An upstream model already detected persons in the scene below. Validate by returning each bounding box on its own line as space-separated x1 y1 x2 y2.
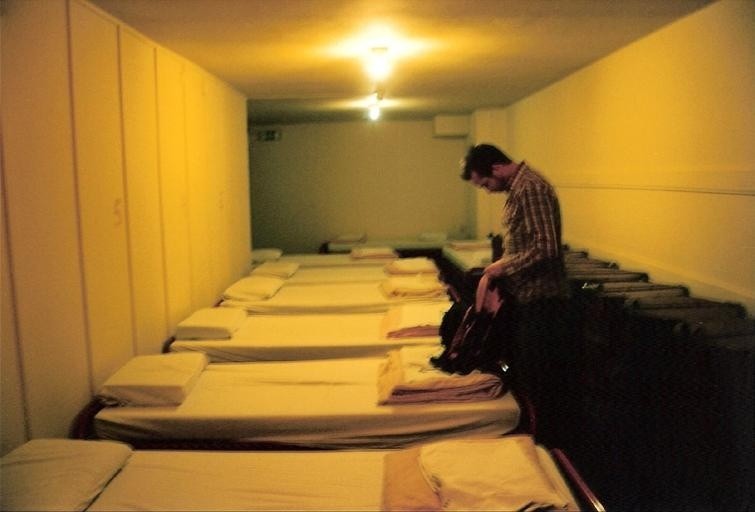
457 142 574 449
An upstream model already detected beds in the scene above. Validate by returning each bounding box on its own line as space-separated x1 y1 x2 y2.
94 344 537 450
251 245 399 268
252 256 441 286
170 301 455 362
219 274 460 315
1 435 603 512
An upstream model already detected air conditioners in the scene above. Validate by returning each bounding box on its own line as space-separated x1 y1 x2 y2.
431 114 470 137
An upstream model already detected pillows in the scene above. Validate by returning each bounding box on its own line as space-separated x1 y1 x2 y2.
174 307 247 340
98 352 211 407
1 439 132 512
251 261 299 279
222 276 283 303
252 248 283 262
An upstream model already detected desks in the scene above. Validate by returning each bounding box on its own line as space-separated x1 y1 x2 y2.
440 244 494 301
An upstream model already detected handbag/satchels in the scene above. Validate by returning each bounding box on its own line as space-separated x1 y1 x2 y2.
430 268 509 375
